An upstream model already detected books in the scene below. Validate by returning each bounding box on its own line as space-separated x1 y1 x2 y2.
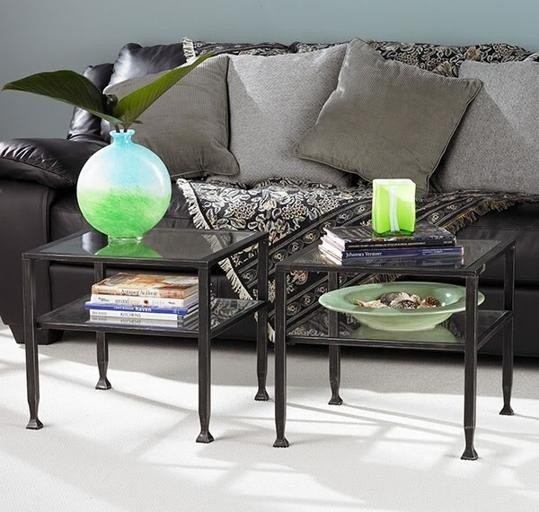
322 224 456 251
320 250 465 268
86 315 220 333
317 237 465 259
84 272 217 321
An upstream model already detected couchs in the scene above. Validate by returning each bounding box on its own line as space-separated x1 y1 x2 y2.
0 40 539 362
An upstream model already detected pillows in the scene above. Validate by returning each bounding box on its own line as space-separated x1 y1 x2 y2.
298 39 482 197
209 44 353 188
100 55 240 180
434 59 539 197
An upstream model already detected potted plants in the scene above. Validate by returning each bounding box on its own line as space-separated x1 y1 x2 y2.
0 53 219 257
